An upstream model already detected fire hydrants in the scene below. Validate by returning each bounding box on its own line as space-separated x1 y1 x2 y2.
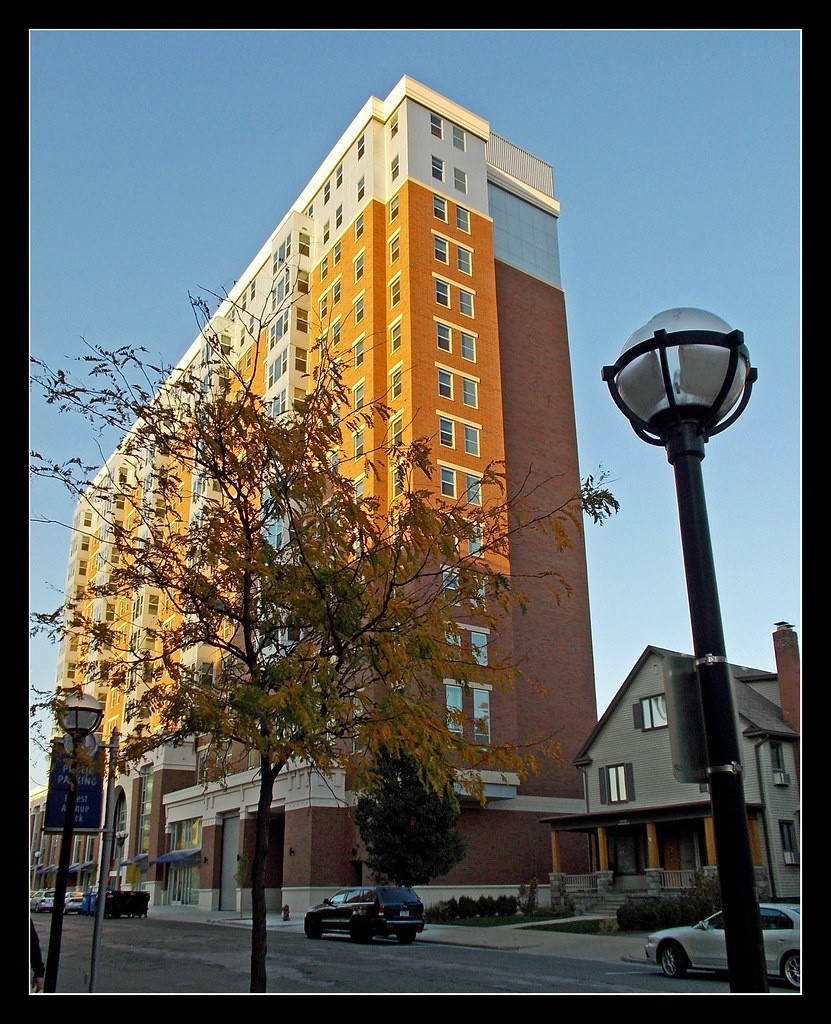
281 904 291 921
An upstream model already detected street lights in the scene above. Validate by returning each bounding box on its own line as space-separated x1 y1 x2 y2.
600 307 772 993
42 691 103 993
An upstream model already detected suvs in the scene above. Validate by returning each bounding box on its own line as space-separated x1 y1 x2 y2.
304 885 426 944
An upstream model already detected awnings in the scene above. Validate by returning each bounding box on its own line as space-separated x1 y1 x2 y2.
68 861 94 873
131 852 149 862
68 862 80 871
29 864 62 875
119 859 132 866
149 848 202 866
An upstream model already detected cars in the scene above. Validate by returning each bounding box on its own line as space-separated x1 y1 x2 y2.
644 903 800 991
30 891 83 915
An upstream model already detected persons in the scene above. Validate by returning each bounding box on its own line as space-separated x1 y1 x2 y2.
29 917 46 993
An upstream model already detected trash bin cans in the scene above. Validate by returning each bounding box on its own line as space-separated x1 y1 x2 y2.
82 891 97 917
112 890 150 918
